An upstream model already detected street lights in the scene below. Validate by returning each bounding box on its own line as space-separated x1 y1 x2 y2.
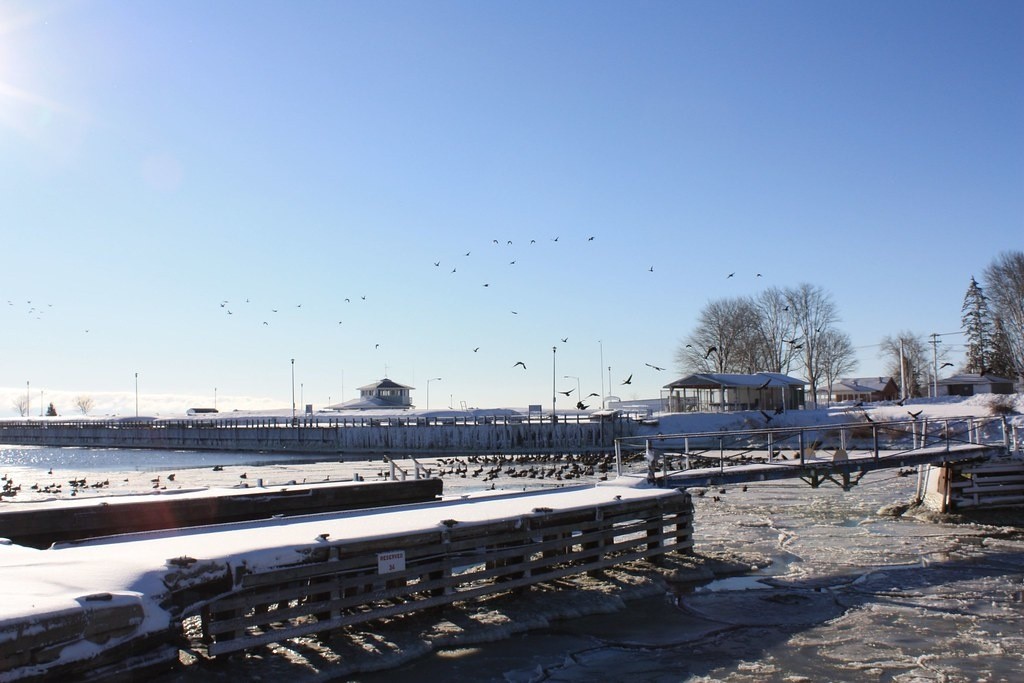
40 391 43 416
291 359 296 418
215 388 216 409
26 381 30 416
564 376 580 402
598 339 604 409
135 372 138 418
427 377 442 409
608 366 611 397
301 383 303 413
553 346 557 424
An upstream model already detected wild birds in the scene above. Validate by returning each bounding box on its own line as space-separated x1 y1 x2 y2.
0 231 959 499
